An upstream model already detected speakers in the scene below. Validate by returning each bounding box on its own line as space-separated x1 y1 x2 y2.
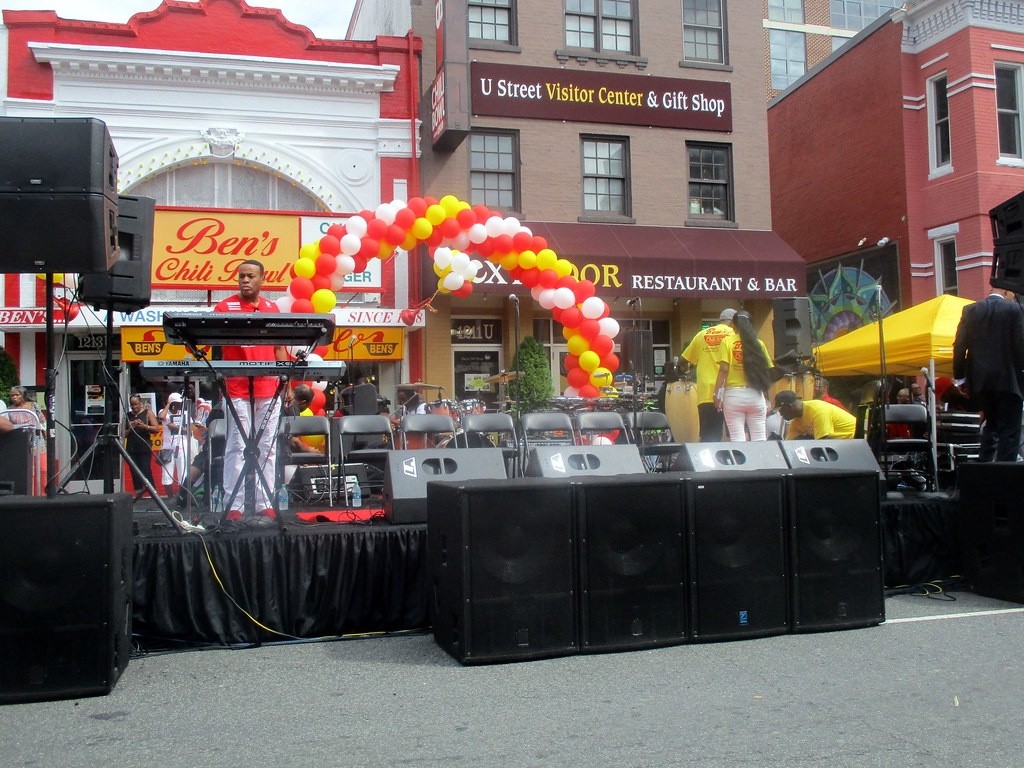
287 439 887 666
989 191 1024 296
337 384 381 450
0 426 33 496
772 297 812 360
956 462 1024 605
620 330 653 375
0 115 158 315
0 492 131 707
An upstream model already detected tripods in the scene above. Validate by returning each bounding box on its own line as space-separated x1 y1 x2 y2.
55 308 187 541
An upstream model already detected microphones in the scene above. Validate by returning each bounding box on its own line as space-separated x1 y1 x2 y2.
626 297 640 308
509 294 519 303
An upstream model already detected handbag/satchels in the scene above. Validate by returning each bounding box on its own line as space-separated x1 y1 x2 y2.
154 449 174 465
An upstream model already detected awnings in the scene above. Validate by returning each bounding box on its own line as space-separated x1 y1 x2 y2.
421 222 809 303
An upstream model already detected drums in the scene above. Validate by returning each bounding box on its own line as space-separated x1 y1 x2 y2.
457 399 486 415
435 432 497 448
425 399 460 429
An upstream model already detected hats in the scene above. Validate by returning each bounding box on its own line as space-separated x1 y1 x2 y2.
771 390 796 411
719 308 738 320
167 392 185 403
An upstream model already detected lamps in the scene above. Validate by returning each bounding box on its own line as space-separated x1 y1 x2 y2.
482 292 488 301
673 299 680 306
401 297 432 326
889 8 907 24
876 237 891 247
53 296 81 322
857 237 867 247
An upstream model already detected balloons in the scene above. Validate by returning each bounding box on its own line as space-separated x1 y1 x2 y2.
273 194 621 447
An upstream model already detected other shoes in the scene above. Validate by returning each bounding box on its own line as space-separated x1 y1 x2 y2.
256 508 277 520
225 509 244 521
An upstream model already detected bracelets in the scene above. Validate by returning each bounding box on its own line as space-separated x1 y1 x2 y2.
713 392 717 396
147 425 149 430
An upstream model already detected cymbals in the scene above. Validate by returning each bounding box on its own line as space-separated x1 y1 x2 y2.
491 400 529 404
395 382 444 390
483 370 526 382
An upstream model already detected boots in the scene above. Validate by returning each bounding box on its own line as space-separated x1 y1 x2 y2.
176 477 195 510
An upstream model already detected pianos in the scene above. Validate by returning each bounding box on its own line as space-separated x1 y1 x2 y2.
138 360 348 535
161 310 337 346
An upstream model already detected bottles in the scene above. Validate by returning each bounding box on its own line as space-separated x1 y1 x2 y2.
212 485 223 513
278 483 288 511
352 480 361 507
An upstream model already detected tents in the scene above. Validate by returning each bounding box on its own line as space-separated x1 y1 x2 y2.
811 293 979 483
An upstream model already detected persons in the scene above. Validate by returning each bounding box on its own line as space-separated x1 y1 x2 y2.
943 379 984 427
119 393 160 498
382 382 430 426
951 283 1024 462
334 376 371 419
276 380 325 488
771 388 857 440
763 397 788 441
348 383 354 387
712 311 774 442
813 378 850 413
0 384 48 497
676 307 737 443
886 381 933 441
158 260 296 522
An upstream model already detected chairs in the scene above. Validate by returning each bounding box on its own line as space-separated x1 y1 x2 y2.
462 413 524 478
279 415 333 507
575 411 630 445
208 418 227 514
856 403 933 479
336 415 395 507
402 413 459 450
519 411 576 476
626 412 683 473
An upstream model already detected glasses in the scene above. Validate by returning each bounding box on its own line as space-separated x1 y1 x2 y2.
129 394 140 400
